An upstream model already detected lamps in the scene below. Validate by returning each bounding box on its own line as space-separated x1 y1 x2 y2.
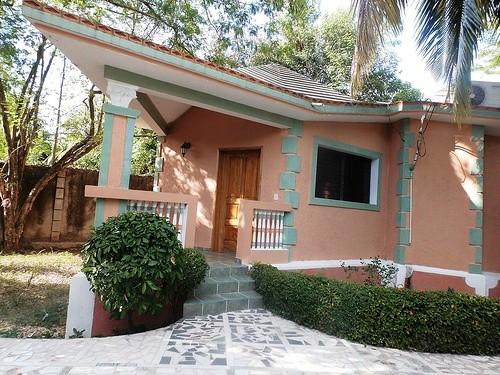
181 140 191 157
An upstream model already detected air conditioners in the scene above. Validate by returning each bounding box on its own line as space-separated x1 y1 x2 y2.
471 81 500 108
